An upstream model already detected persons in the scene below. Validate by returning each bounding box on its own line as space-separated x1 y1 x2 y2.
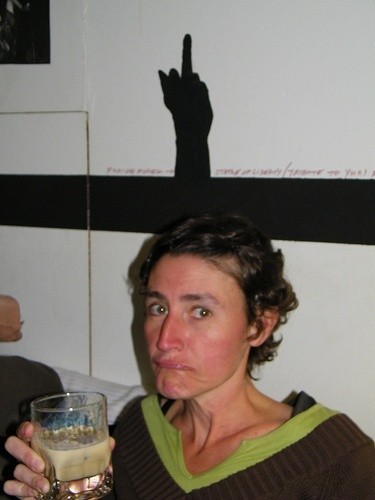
3 210 375 500
1 292 68 499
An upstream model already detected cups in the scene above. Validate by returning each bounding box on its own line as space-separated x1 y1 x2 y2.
30 392 114 500
